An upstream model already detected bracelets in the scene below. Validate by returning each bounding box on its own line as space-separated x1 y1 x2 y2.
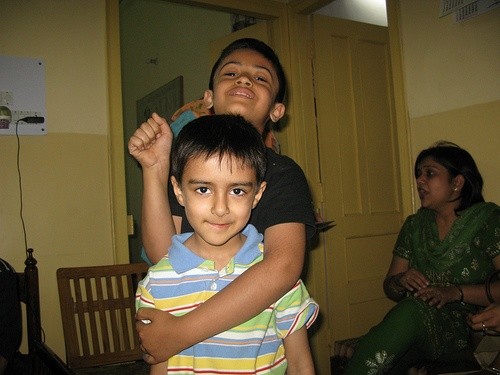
457 285 463 305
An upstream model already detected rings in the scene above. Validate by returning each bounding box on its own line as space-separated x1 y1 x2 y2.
482 322 488 332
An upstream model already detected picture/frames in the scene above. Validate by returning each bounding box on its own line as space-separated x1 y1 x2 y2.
135 76 183 169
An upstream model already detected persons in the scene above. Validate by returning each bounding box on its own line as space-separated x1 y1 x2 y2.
349 141 500 375
134 113 320 375
128 38 317 366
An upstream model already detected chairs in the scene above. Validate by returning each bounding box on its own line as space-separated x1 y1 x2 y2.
0 249 73 375
56 261 150 375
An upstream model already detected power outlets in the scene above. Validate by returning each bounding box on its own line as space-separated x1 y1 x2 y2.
0 90 13 106
10 110 41 126
